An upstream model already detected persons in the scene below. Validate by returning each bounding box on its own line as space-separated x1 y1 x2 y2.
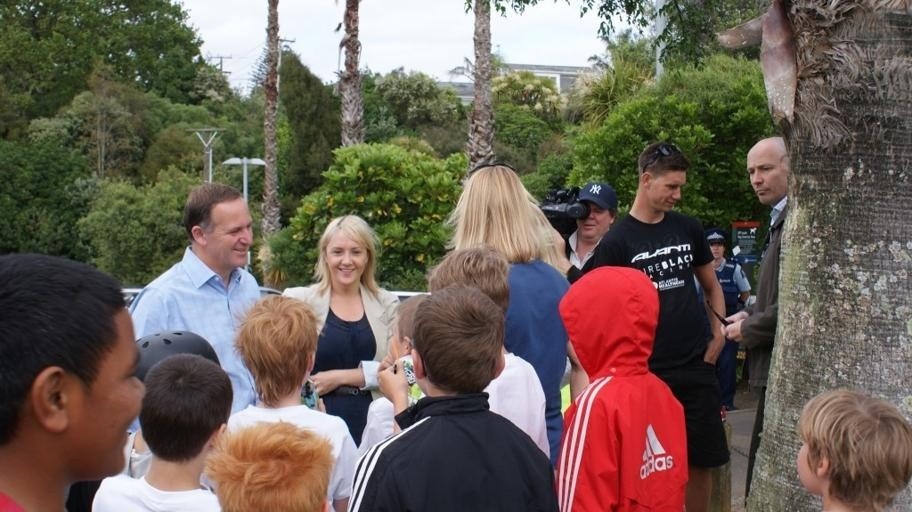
201 295 359 512
797 390 912 512
592 143 730 512
281 215 411 443
704 230 752 413
559 182 618 285
357 294 428 461
119 330 221 495
125 183 270 434
443 162 589 479
0 254 146 512
556 266 689 512
90 353 233 512
429 244 551 459
720 135 790 507
210 425 330 512
346 282 560 512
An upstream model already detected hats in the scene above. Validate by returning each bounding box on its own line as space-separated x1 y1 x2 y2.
579 182 617 211
704 228 728 244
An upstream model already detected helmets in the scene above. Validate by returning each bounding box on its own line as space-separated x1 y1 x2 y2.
133 330 220 382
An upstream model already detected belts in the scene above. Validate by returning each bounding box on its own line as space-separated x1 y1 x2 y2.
333 385 370 397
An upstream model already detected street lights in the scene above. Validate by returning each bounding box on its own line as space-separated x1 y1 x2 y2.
224 155 270 233
221 157 266 267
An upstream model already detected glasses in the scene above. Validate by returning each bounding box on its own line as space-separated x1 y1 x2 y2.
643 143 682 171
704 299 731 327
467 159 515 175
